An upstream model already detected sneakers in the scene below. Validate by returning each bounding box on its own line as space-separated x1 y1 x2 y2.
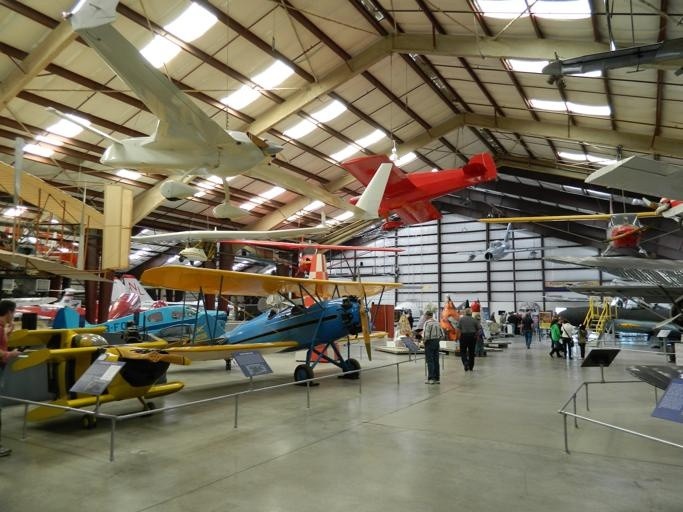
550 352 563 358
425 379 440 383
463 361 473 371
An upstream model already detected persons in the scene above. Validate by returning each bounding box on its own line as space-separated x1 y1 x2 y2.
520 313 536 349
490 308 531 336
474 313 485 357
408 313 413 328
422 310 444 384
0 300 23 458
577 325 587 360
561 319 576 360
549 317 564 358
457 307 480 372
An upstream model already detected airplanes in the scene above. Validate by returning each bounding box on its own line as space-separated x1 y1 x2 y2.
476 194 683 369
